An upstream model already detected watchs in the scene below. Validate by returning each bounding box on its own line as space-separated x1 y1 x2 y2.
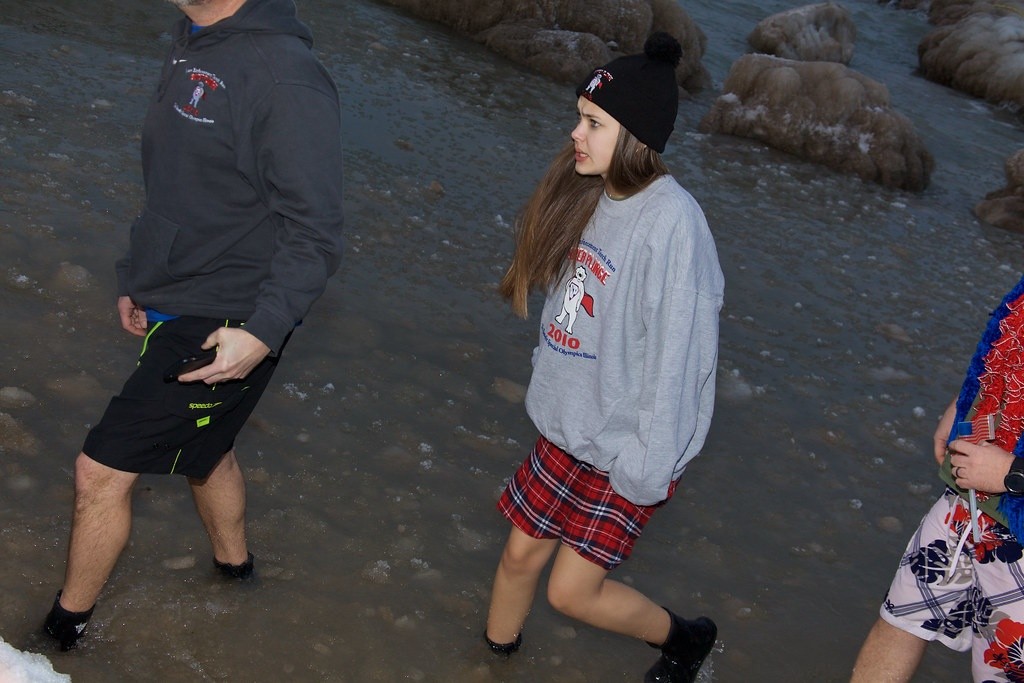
1003 456 1024 497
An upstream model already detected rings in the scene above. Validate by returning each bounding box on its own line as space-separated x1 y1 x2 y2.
956 467 963 478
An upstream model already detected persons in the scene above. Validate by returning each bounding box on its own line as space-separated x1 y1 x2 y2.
850 266 1023 682
24 0 345 653
480 32 723 682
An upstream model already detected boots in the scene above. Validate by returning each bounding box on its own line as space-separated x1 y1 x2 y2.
212 554 256 584
645 606 718 683
484 630 522 657
40 589 98 652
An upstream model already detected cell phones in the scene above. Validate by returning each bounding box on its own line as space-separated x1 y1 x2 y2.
162 350 217 383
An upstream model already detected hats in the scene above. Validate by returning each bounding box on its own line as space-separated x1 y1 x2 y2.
575 32 683 155
